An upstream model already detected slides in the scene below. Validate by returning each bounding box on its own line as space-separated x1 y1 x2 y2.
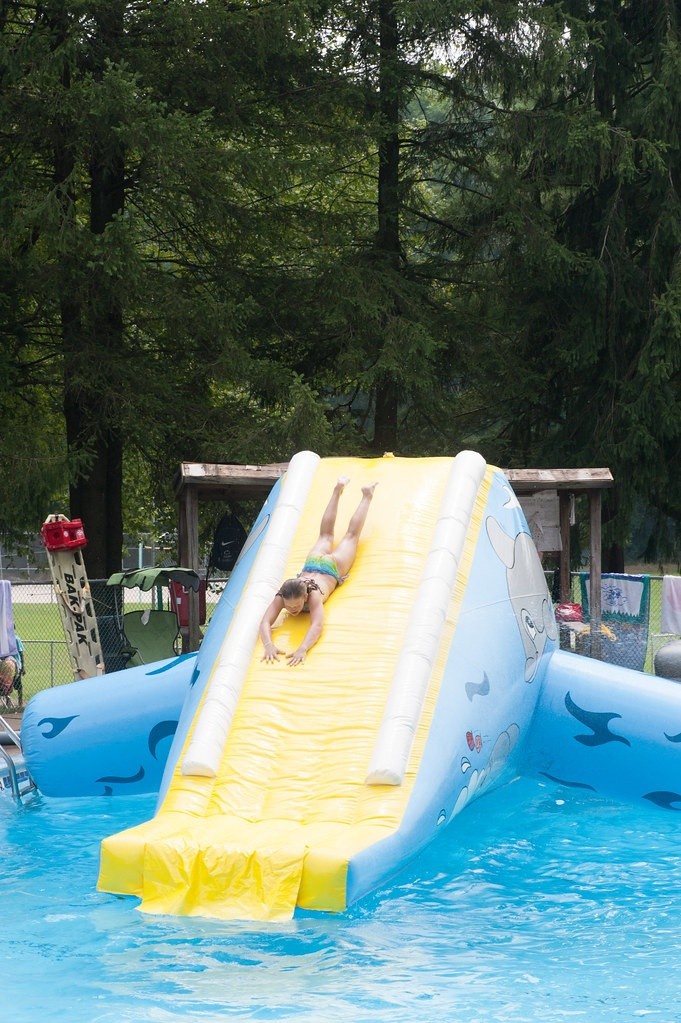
93 450 497 928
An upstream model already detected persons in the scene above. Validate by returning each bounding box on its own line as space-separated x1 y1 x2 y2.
0 623 26 707
259 475 380 667
